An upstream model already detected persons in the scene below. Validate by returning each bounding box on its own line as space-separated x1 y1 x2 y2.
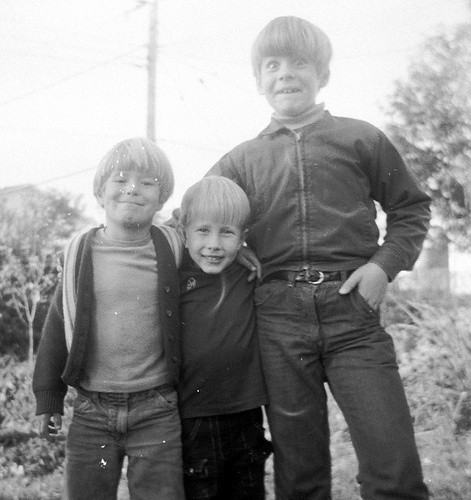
33 139 260 500
164 17 432 500
176 175 274 500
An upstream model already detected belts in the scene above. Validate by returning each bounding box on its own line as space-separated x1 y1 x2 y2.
264 270 357 285
